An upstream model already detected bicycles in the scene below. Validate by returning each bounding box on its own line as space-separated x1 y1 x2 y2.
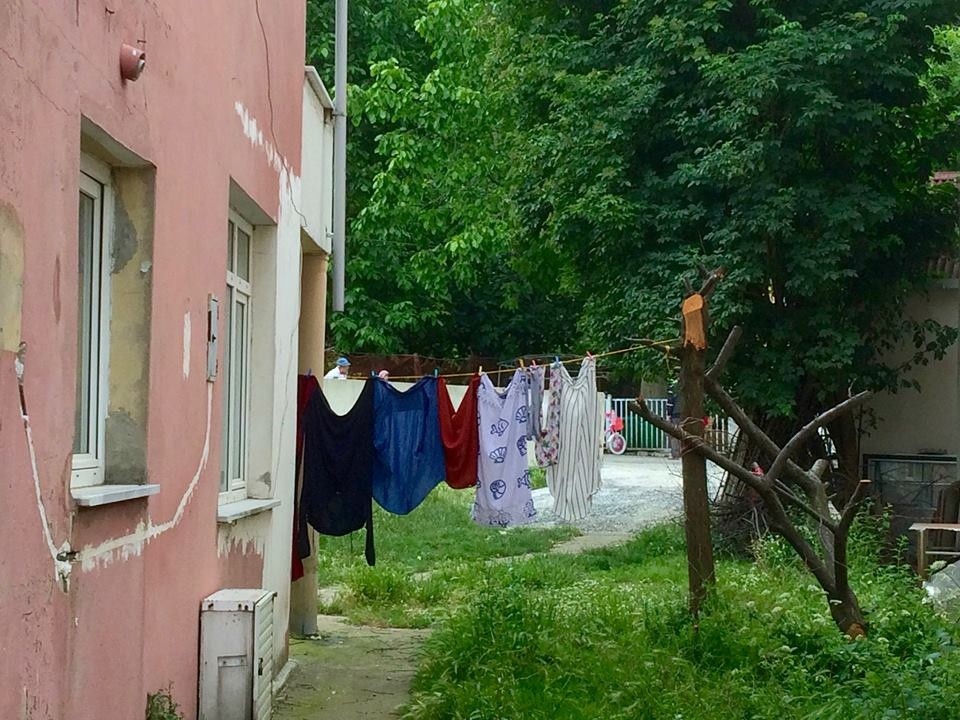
604 409 627 455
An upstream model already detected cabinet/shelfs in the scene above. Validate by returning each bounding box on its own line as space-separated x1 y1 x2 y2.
199 589 277 720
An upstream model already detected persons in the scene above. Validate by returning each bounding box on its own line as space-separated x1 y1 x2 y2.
325 359 349 377
667 370 682 461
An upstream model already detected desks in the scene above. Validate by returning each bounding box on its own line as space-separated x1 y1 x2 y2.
908 523 960 588
